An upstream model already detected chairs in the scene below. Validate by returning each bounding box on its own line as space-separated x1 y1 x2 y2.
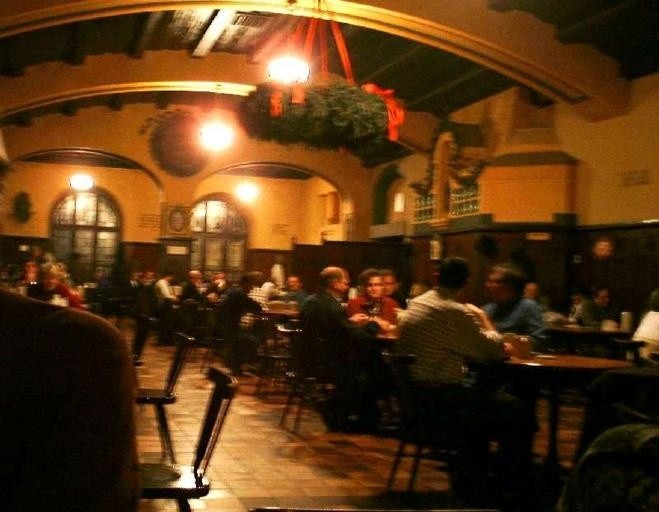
83 285 160 367
240 281 398 434
137 367 239 512
384 311 659 512
134 332 195 464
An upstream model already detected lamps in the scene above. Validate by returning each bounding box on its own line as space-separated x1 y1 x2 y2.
194 81 237 157
259 0 314 86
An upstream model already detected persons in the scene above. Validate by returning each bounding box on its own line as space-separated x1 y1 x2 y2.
0 140 140 512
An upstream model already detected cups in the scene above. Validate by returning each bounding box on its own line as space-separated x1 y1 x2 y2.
620 311 634 333
513 335 536 360
501 333 518 344
59 298 69 307
16 286 27 295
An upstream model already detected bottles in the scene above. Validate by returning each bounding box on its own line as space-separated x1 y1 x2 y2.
411 180 478 222
51 294 60 306
